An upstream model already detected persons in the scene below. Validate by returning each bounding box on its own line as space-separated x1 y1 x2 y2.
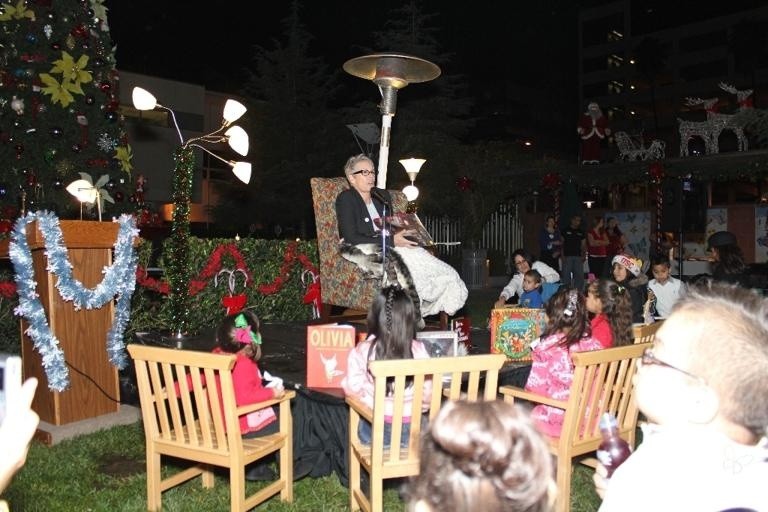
577 101 613 168
335 154 470 320
405 397 557 510
342 283 436 447
491 210 750 443
592 279 768 511
158 311 284 482
0 355 43 512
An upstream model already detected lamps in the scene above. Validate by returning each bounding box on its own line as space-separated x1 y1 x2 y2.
399 159 426 202
65 180 103 220
131 86 252 186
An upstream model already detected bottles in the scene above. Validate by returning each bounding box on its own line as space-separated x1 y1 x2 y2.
597 411 633 479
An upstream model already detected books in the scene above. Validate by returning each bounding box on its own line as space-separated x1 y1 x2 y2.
304 323 356 390
373 212 462 247
415 327 461 384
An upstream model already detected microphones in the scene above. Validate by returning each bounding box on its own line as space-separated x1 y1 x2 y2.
370 187 389 206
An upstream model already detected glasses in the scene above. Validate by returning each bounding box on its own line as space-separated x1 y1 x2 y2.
353 169 379 176
641 345 694 378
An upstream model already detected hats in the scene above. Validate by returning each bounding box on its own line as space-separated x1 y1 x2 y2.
611 253 643 278
706 231 737 252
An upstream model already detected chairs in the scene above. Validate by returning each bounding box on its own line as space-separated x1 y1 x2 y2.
311 176 448 330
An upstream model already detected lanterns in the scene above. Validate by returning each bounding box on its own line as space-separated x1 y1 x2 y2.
456 176 472 193
543 170 558 190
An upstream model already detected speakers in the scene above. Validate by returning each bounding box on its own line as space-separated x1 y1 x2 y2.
663 179 707 228
